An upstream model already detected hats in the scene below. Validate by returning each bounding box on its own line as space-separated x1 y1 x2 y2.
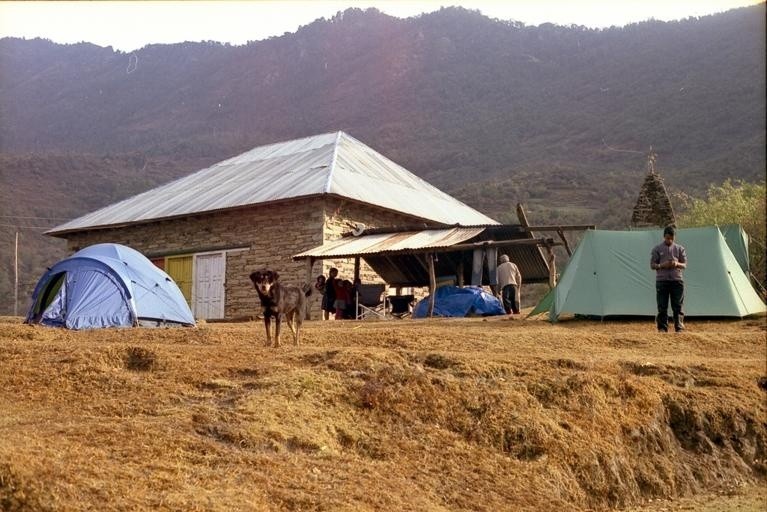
318 275 325 282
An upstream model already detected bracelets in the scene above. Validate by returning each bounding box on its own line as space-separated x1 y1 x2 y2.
658 264 661 268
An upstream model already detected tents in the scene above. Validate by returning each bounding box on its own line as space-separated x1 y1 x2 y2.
523 222 767 323
22 242 196 328
411 283 506 319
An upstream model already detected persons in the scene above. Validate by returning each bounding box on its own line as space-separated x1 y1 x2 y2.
315 267 354 321
493 254 522 314
649 226 688 333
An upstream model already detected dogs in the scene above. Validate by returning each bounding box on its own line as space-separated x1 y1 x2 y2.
249 269 313 347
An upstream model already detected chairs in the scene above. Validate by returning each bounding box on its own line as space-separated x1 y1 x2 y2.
383 292 417 320
351 280 386 322
